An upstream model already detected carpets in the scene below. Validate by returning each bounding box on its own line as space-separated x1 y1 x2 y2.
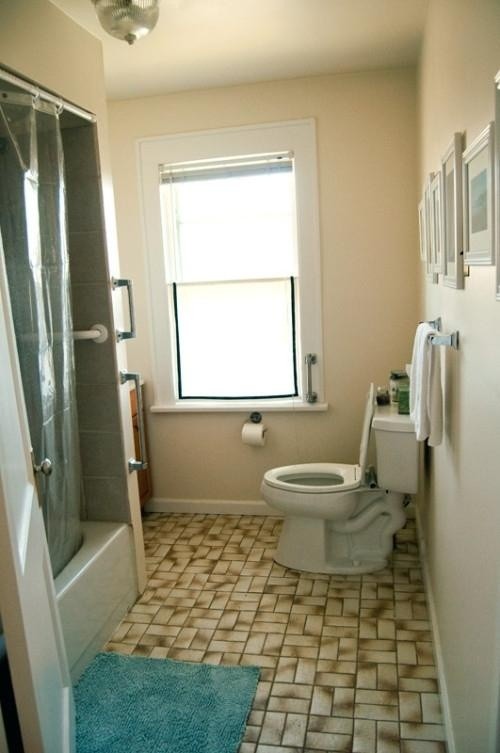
72 649 262 752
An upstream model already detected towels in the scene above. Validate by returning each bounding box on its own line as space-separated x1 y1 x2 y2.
409 321 444 449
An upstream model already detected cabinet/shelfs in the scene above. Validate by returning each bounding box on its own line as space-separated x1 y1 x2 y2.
128 381 154 507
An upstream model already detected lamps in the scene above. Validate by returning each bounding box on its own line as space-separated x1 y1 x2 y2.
89 0 163 47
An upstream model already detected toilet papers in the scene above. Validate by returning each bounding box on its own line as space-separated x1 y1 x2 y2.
241 423 266 447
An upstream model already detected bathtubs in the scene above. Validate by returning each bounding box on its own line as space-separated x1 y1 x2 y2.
46 520 140 685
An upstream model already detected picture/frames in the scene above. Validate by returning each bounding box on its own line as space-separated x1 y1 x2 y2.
416 121 500 300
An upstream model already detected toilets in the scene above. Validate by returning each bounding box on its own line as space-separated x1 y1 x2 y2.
260 381 421 575
